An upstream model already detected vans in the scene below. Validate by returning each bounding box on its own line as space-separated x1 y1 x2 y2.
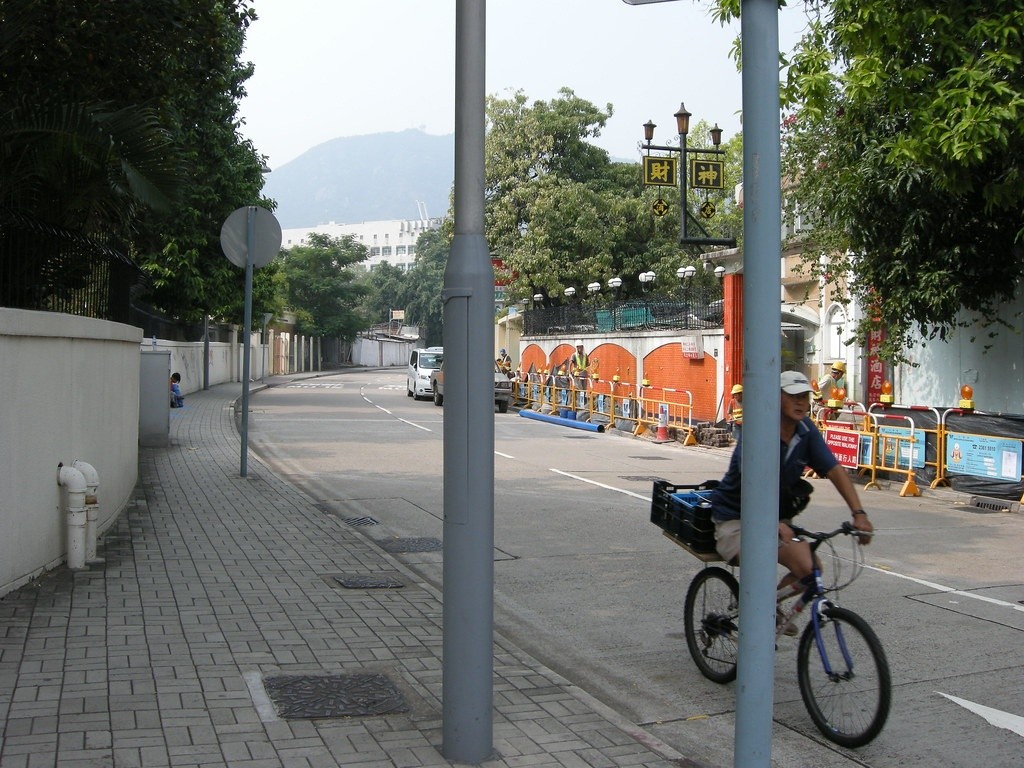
407 347 444 400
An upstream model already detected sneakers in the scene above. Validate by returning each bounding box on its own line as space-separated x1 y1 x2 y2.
776 608 799 637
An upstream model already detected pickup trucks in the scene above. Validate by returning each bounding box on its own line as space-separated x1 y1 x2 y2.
430 356 512 414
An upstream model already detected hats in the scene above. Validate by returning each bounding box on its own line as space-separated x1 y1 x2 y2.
500 349 505 354
780 370 817 398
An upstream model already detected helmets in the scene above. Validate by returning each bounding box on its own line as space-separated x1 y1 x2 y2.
576 340 583 346
730 384 743 394
832 361 846 374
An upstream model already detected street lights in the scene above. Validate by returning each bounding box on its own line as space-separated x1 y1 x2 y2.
588 281 601 329
642 100 730 242
607 278 622 326
676 266 696 330
638 271 656 324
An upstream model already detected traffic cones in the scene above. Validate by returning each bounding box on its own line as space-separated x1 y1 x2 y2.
652 405 675 444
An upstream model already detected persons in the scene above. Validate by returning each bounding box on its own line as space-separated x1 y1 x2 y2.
727 384 743 442
812 360 856 421
170 373 181 391
497 348 512 371
712 370 872 637
568 340 590 404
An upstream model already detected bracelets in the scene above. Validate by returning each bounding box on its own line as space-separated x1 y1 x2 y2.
852 509 865 516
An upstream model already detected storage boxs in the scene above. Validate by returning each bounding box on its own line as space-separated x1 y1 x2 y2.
651 476 720 554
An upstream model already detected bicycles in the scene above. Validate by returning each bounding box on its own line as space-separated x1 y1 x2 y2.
683 520 893 751
